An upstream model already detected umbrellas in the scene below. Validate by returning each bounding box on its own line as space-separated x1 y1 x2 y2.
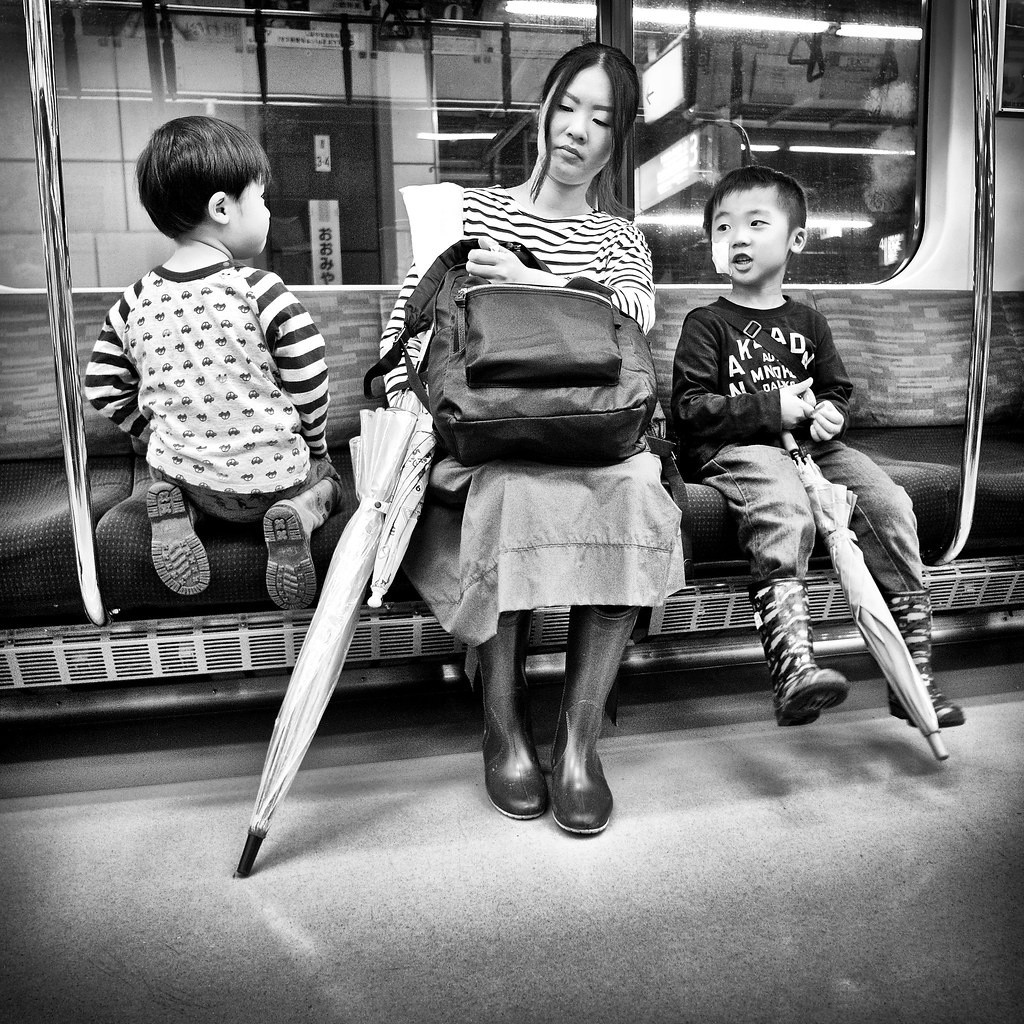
782 429 949 760
368 369 436 609
236 374 437 878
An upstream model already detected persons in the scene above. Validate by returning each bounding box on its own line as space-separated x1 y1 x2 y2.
82 115 343 610
672 167 966 728
379 42 687 835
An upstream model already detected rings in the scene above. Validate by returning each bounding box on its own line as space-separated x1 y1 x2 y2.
488 243 500 253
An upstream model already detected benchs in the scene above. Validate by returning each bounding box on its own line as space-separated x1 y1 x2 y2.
1 280 1024 724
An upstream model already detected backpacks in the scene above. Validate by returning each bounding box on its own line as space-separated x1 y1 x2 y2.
364 237 658 467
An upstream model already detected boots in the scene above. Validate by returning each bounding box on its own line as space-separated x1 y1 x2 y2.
550 605 642 833
479 610 549 819
263 477 337 609
883 590 965 728
147 481 211 595
749 576 849 727
